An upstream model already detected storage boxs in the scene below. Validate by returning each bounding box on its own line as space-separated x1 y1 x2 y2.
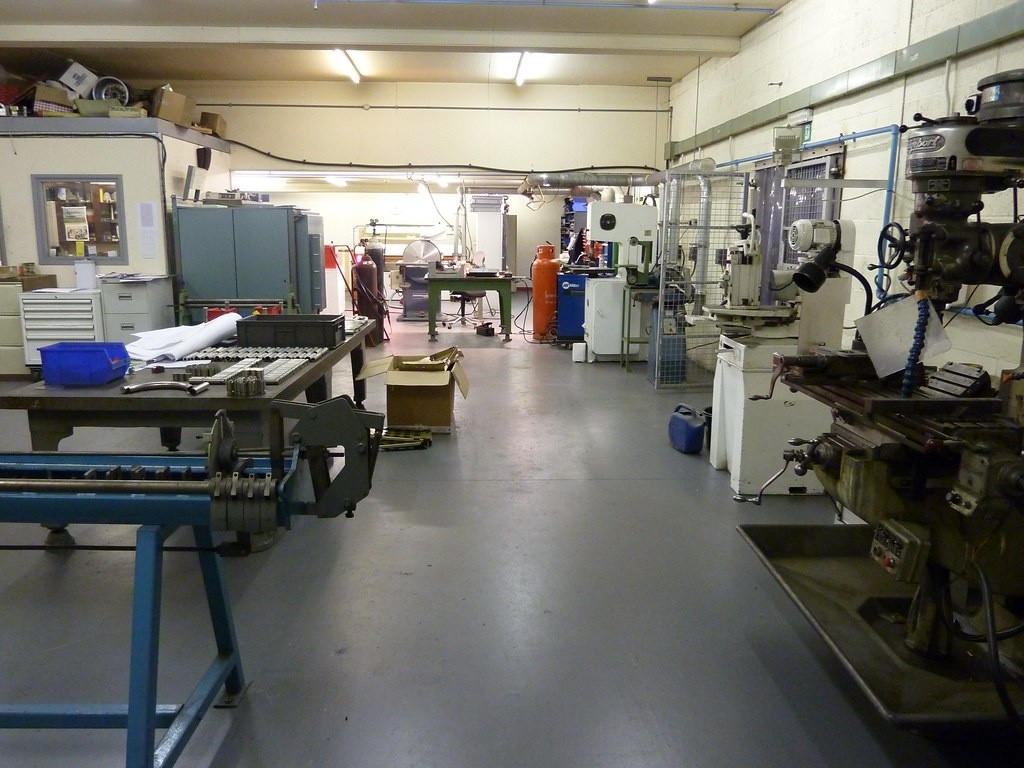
37 340 130 385
22 49 228 140
356 355 468 434
236 315 346 349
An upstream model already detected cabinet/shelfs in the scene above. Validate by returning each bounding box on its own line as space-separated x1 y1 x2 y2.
0 282 33 376
100 277 173 345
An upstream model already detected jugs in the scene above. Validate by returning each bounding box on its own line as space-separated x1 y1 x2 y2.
668 403 706 454
